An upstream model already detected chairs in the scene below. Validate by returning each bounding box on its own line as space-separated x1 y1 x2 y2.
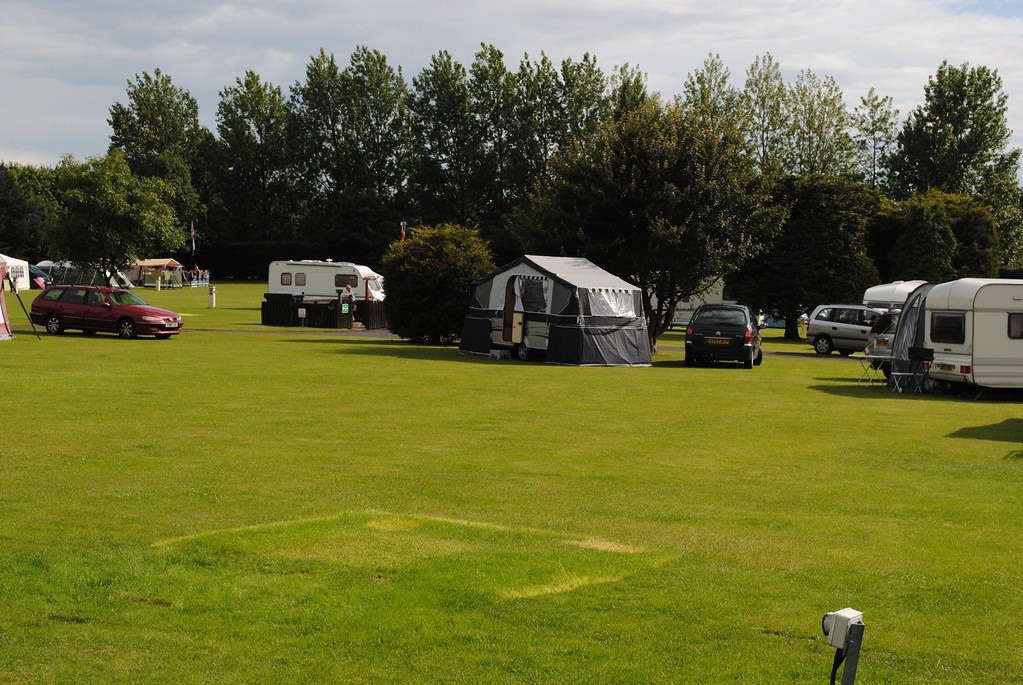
861 314 877 326
90 295 99 305
891 347 935 393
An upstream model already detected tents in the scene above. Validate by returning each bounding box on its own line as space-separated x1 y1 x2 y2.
887 282 946 393
0 256 14 341
0 254 30 290
458 254 653 367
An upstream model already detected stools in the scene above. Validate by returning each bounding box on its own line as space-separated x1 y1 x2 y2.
488 349 511 361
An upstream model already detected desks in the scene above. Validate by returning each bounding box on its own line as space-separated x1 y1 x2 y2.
849 354 895 388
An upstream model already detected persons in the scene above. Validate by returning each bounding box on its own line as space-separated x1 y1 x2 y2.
344 285 356 321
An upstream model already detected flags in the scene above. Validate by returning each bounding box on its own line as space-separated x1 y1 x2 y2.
191 223 194 237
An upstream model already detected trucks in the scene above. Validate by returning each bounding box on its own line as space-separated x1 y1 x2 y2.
268 258 386 303
862 279 928 310
923 278 1023 401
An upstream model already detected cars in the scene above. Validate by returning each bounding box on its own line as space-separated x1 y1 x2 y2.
29 284 184 340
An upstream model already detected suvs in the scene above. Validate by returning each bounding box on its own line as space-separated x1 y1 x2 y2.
29 264 54 289
806 304 887 356
684 301 768 369
864 308 903 379
